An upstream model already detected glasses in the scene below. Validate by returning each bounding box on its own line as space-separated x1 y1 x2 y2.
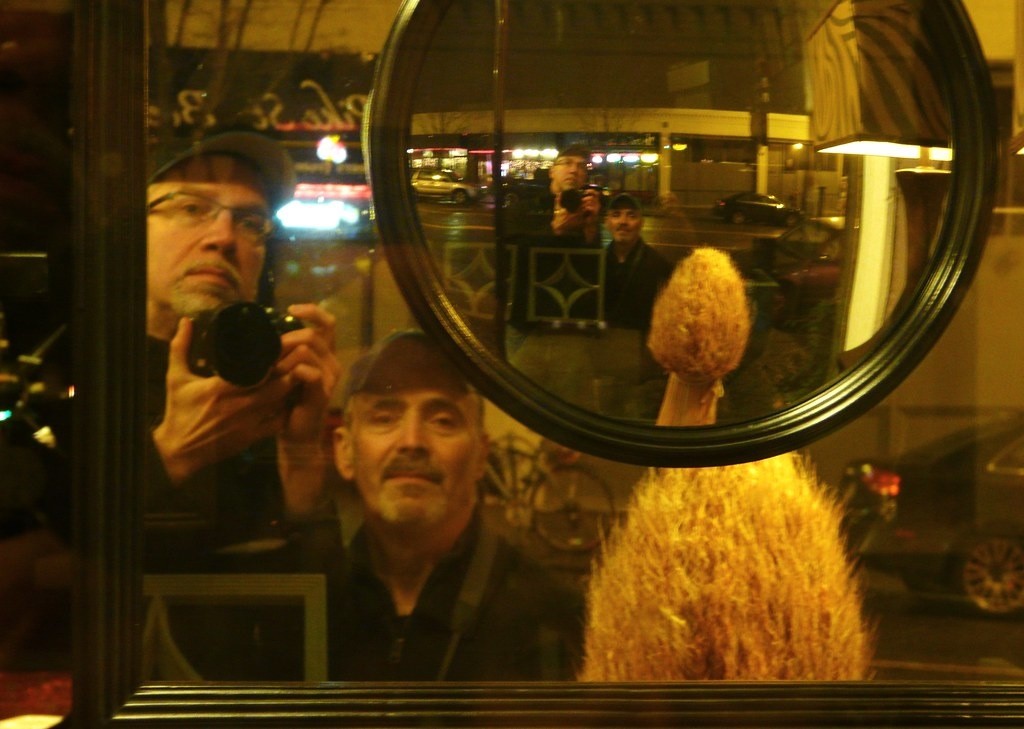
150 190 278 244
558 158 588 170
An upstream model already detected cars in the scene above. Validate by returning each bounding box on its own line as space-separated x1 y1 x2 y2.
735 215 846 310
842 412 1024 616
411 169 477 207
713 189 804 228
502 183 553 210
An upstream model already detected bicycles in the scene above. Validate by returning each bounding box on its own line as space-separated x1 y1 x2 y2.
483 432 618 553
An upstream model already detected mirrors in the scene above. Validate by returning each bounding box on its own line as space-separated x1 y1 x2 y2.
366 0 998 469
67 0 1024 729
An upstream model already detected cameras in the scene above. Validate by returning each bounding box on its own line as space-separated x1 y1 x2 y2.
181 298 305 388
560 188 588 213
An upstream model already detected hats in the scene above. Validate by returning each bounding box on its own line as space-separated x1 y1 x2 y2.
342 326 478 403
555 143 592 164
146 130 297 217
609 192 642 212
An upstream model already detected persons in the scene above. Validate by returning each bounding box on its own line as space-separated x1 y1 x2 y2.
231 327 590 680
110 125 345 682
506 141 605 328
568 193 676 327
